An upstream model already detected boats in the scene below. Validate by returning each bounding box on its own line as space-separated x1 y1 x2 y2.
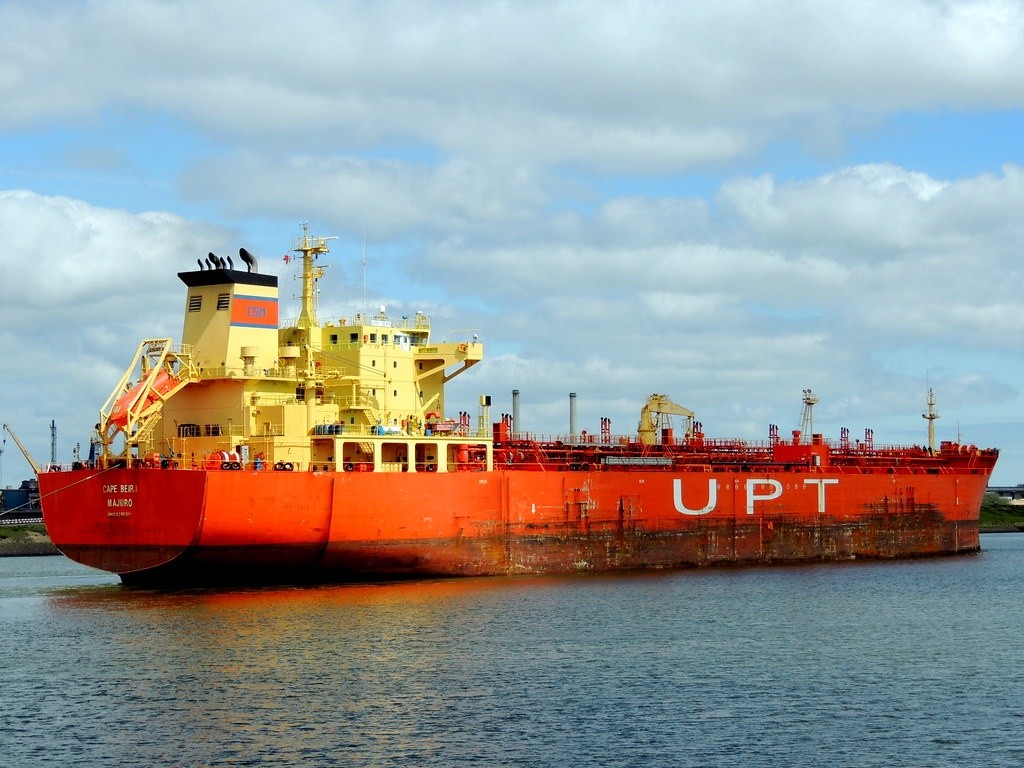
36 220 1000 583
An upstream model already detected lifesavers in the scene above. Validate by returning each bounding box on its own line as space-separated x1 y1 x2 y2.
275 462 294 471
571 464 588 470
222 462 240 470
742 466 747 471
928 469 937 473
427 465 433 471
347 463 353 471
888 469 892 473
49 465 61 472
161 460 168 468
459 344 467 353
73 462 82 469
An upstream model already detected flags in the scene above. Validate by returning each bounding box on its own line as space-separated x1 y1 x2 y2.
283 254 291 264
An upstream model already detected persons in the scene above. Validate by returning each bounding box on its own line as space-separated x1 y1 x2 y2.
928 446 933 457
922 444 928 454
